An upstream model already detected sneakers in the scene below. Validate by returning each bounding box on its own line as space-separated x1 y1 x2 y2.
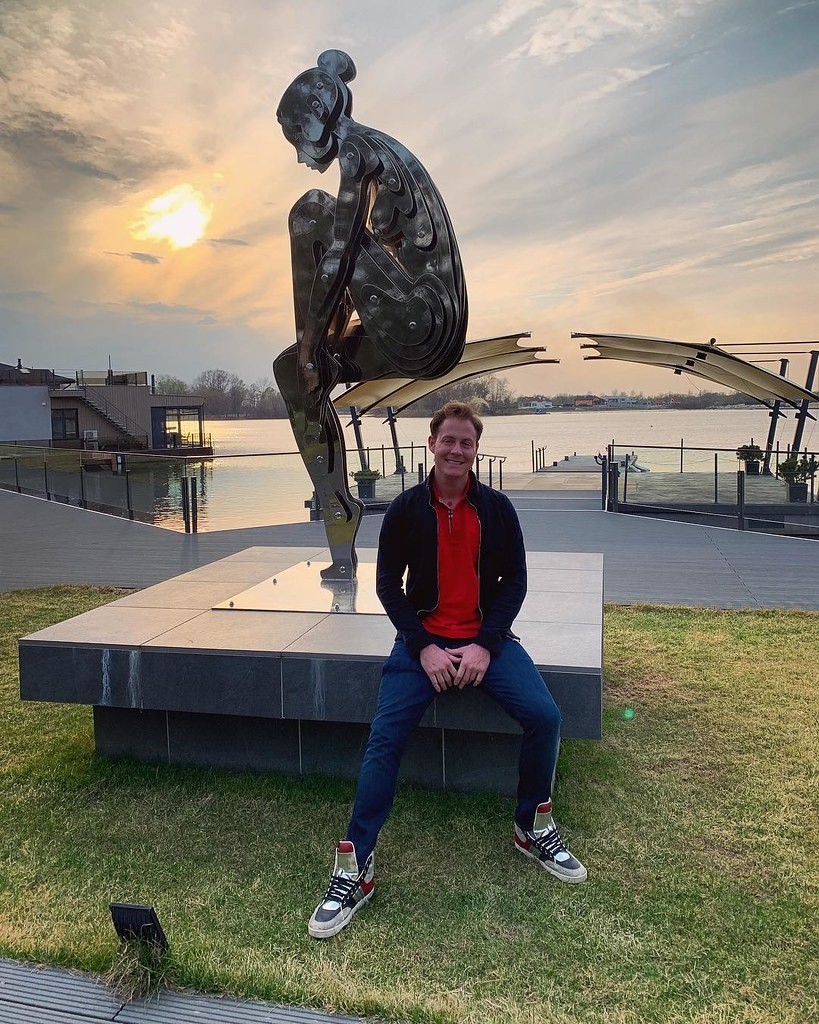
308 841 375 938
514 798 588 884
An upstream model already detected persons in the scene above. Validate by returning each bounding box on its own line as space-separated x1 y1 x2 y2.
303 404 589 940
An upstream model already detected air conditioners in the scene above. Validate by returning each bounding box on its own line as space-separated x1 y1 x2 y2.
84 430 98 441
84 442 98 450
